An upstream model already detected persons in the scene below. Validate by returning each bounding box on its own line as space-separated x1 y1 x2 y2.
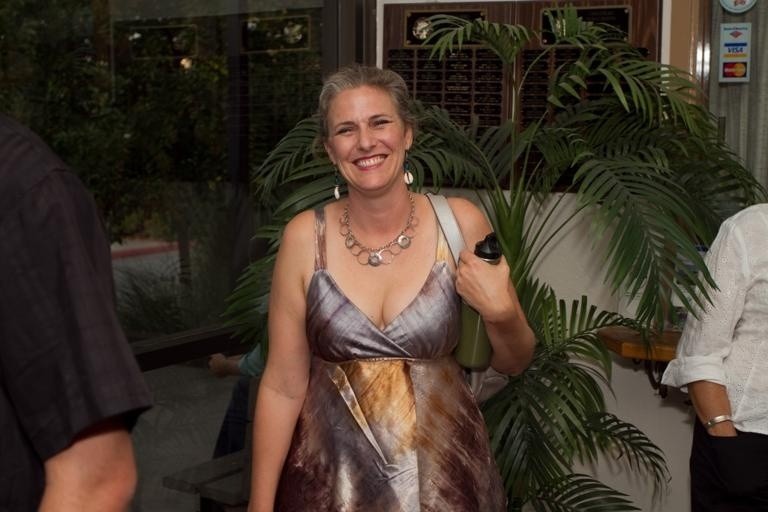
200 61 537 512
660 203 768 512
0 114 155 512
208 322 269 377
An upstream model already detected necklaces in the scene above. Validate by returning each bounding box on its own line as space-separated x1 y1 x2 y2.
338 191 420 267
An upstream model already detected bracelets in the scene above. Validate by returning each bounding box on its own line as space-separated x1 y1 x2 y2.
707 414 734 427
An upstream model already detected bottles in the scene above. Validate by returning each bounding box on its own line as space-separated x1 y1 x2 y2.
457 234 504 373
